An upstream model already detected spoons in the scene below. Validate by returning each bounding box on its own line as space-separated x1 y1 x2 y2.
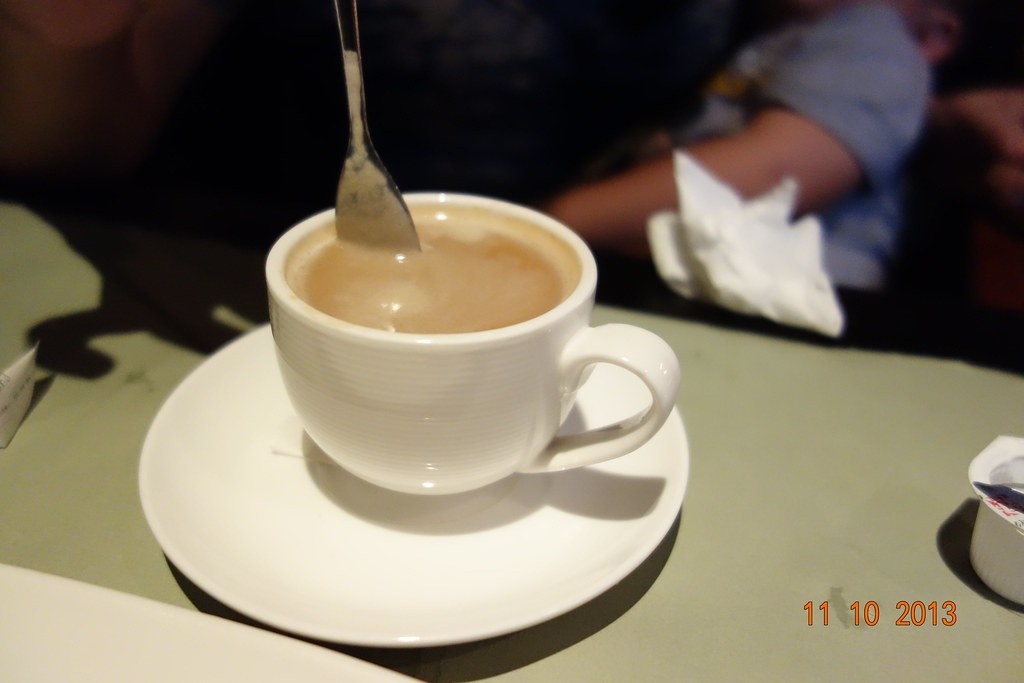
335 0 422 252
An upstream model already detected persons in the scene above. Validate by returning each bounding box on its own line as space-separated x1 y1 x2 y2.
0 0 932 349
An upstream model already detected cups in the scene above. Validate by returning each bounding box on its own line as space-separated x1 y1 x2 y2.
264 192 681 495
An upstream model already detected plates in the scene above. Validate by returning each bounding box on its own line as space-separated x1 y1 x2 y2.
138 322 690 648
